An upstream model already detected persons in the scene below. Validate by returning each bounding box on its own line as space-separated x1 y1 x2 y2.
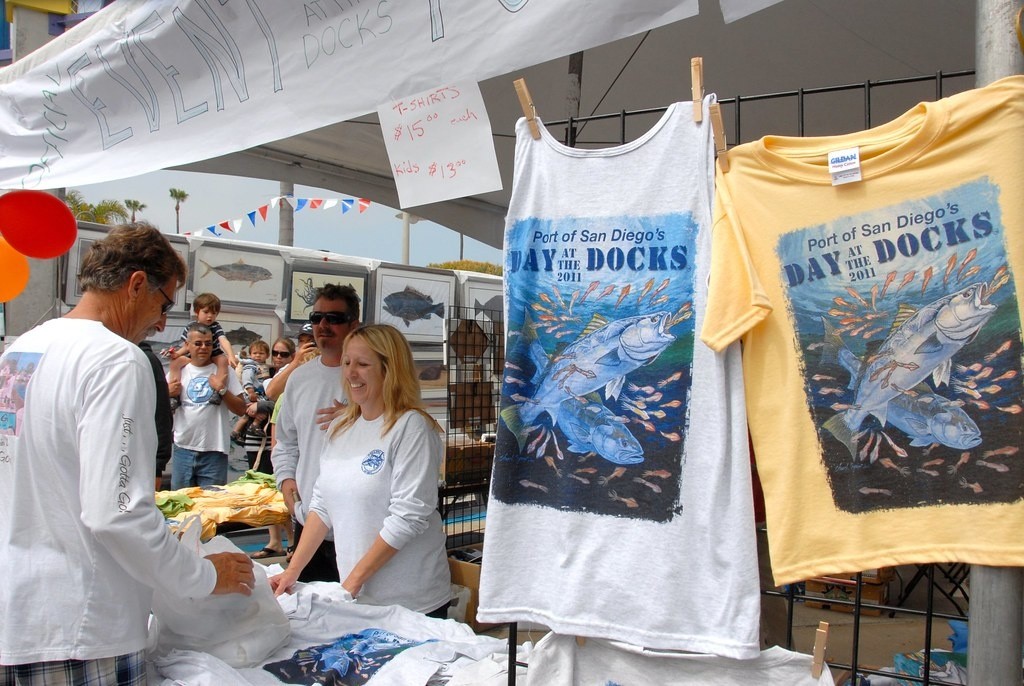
271 282 360 584
140 293 317 491
268 323 453 620
0 222 255 686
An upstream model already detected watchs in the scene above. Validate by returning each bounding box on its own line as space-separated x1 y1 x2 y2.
219 386 228 395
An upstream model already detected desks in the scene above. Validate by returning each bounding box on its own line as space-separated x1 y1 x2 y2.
437 480 490 520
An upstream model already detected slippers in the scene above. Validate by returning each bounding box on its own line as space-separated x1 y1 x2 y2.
250 547 287 559
287 545 294 563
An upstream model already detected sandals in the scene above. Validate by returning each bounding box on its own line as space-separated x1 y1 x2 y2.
230 430 245 446
247 424 266 437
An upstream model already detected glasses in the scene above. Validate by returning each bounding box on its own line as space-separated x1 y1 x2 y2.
187 339 212 346
272 349 292 358
145 277 175 315
308 311 355 325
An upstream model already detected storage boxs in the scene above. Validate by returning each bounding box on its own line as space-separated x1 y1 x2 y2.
803 566 896 617
445 542 510 634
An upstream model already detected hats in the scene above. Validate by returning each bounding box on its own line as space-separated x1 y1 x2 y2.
298 322 313 336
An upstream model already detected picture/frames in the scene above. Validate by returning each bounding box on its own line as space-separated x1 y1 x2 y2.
56 221 504 399
